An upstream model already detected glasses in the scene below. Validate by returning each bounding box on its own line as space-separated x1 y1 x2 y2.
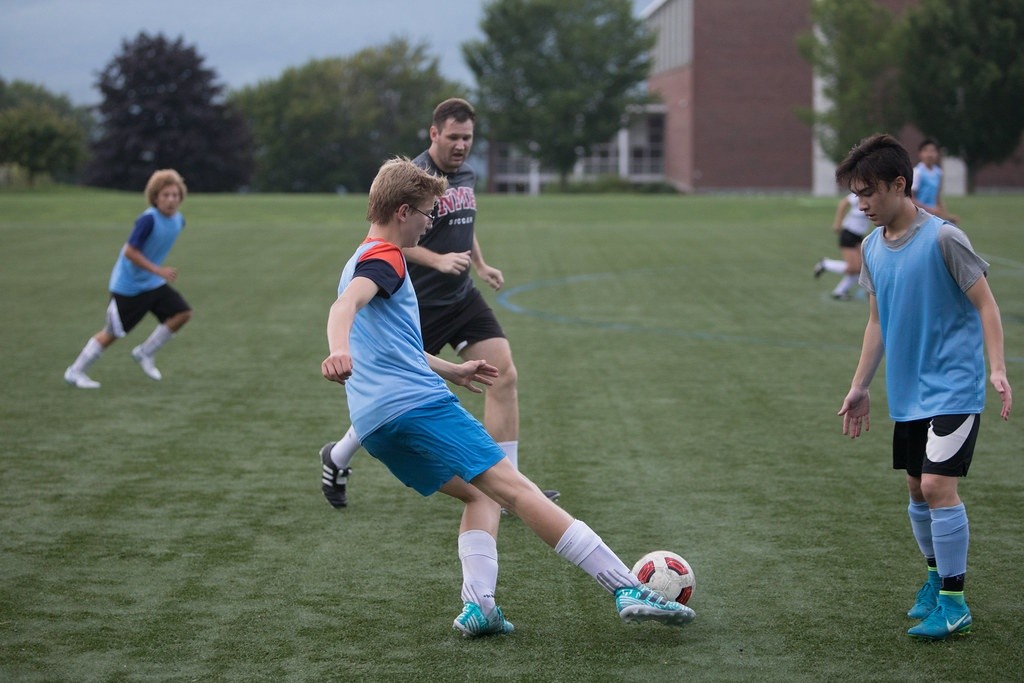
409 205 435 224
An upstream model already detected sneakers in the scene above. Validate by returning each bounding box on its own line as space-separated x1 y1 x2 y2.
319 441 352 508
501 490 561 515
615 584 696 626
452 603 514 636
908 571 945 619
908 595 972 639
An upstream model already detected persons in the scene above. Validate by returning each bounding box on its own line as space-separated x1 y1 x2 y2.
318 99 562 518
63 168 191 388
813 189 871 303
909 139 959 225
318 158 696 634
833 132 1012 641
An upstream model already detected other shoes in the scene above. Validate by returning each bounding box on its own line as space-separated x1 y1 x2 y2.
64 366 101 388
814 257 825 277
132 345 161 381
834 294 850 302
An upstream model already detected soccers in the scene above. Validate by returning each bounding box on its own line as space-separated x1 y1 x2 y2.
632 550 696 606
